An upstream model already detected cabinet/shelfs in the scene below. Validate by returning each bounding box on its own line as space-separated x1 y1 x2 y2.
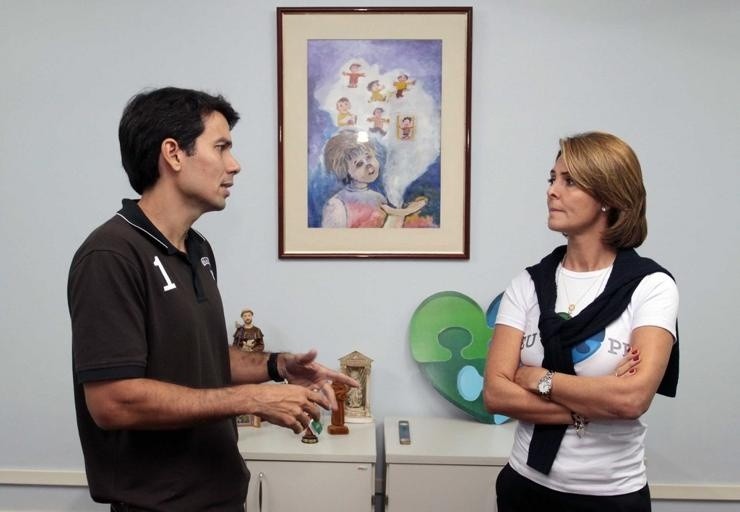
381 411 526 511
236 420 376 509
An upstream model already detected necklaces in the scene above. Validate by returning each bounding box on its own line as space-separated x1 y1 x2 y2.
559 251 612 317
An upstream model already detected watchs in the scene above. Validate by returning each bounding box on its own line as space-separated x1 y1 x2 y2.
538 369 557 400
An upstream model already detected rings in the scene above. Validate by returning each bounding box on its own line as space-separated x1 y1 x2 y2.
311 388 319 393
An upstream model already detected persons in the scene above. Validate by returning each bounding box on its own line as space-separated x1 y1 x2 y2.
480 130 681 512
64 86 363 511
232 307 266 353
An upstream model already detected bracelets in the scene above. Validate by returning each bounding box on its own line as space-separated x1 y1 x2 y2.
572 411 591 437
266 352 285 383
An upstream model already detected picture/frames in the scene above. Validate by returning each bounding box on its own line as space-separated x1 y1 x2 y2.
276 5 474 263
